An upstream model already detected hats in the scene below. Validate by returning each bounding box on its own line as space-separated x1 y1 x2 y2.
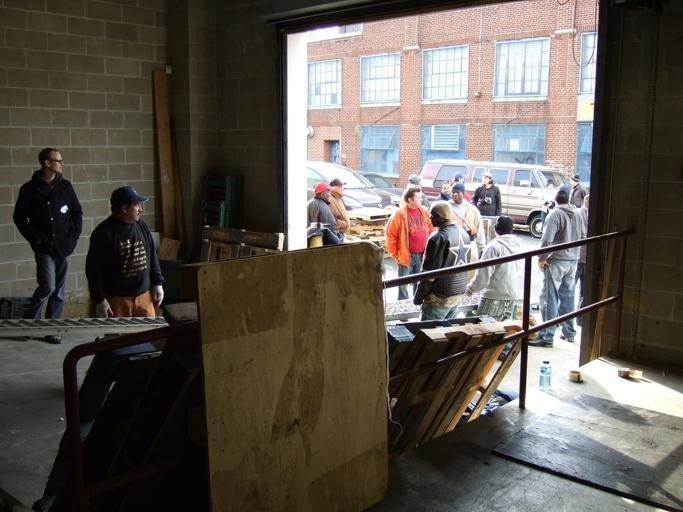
482 172 492 178
571 175 581 182
454 172 465 180
555 183 572 203
110 186 150 204
451 183 466 194
409 174 421 183
330 178 347 186
314 182 335 194
431 200 452 221
494 216 513 234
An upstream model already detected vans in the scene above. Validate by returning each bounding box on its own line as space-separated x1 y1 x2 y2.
418 160 569 240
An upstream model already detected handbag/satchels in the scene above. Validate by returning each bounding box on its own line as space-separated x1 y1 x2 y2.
470 226 478 236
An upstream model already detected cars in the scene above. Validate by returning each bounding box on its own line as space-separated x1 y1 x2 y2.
308 161 429 257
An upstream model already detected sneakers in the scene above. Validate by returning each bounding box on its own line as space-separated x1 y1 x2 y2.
561 333 575 343
45 334 62 345
528 334 553 348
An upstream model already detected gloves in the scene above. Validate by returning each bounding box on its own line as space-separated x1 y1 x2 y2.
152 284 164 309
95 298 114 318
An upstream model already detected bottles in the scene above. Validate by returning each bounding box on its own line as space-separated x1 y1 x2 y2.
538 360 552 392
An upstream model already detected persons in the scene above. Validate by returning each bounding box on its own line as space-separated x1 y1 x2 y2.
568 176 586 209
452 173 466 184
528 184 585 346
385 185 437 301
329 178 350 243
573 194 590 327
540 179 559 231
308 182 339 236
407 174 430 212
437 182 453 202
85 185 166 318
448 182 486 283
13 148 83 321
413 200 472 321
466 217 523 322
472 173 502 240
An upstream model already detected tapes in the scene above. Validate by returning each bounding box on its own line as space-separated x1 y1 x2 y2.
569 370 582 382
618 367 643 378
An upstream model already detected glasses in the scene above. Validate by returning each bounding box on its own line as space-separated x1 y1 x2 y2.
48 159 64 162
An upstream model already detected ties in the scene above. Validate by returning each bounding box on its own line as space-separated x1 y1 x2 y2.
571 189 577 199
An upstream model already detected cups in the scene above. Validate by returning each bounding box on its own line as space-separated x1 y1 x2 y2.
569 370 583 382
618 367 630 378
